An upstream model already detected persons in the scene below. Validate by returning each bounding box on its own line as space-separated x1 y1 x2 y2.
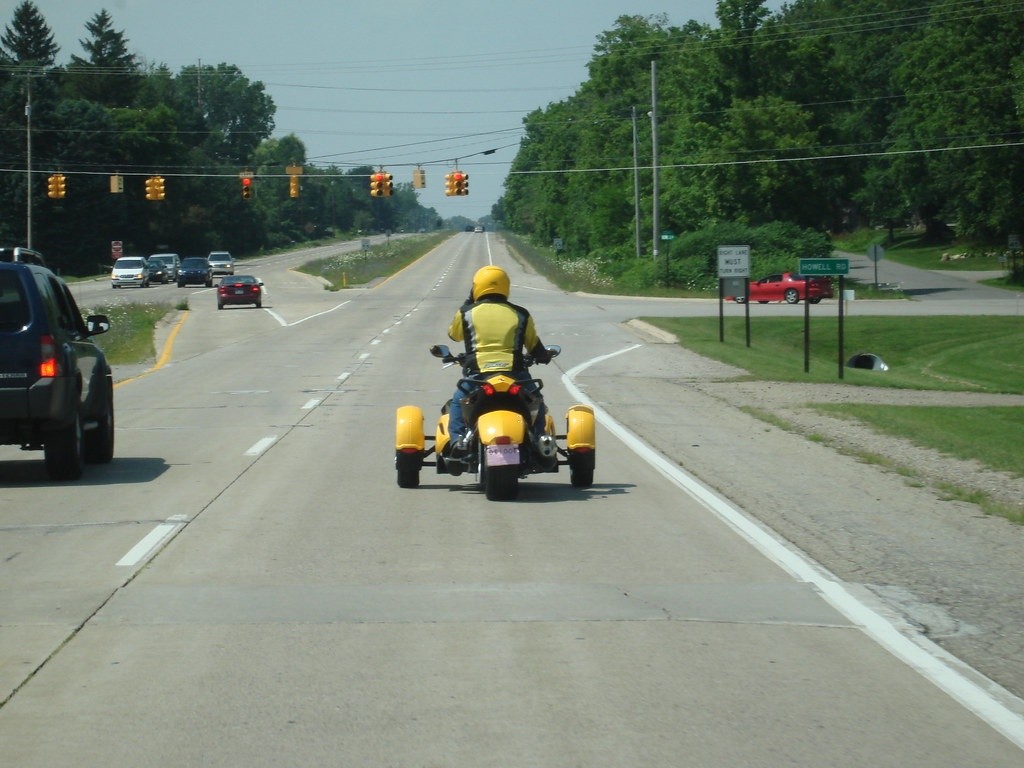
449 266 556 459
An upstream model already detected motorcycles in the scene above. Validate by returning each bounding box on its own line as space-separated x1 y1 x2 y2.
395 345 596 502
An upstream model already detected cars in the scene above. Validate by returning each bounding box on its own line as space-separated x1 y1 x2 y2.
215 275 264 309
465 226 485 233
725 272 834 304
148 253 181 282
418 228 426 233
146 259 169 285
110 257 150 289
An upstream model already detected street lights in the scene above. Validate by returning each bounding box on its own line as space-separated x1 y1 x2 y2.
648 61 659 264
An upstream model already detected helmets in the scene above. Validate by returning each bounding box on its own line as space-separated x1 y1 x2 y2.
473 266 509 302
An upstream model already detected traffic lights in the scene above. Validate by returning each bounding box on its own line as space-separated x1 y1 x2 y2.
242 178 251 199
370 173 393 198
414 170 426 189
144 176 166 201
445 170 469 197
110 176 123 193
47 174 67 199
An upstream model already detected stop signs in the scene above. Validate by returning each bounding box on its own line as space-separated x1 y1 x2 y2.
112 241 123 252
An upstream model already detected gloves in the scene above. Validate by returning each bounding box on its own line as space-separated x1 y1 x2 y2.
466 288 473 304
530 340 552 365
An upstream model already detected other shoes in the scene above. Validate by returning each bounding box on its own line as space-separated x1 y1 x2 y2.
447 442 464 476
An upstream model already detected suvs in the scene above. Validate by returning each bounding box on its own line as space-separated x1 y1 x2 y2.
0 247 114 481
207 251 236 275
176 257 213 288
